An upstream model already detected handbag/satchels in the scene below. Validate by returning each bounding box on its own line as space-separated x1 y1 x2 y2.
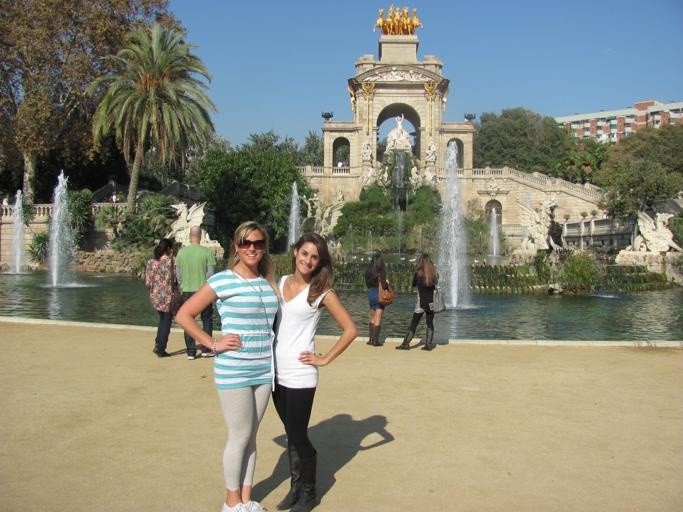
170 292 182 316
432 289 445 313
378 282 392 305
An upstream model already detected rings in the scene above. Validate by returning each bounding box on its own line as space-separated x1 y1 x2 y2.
312 352 315 355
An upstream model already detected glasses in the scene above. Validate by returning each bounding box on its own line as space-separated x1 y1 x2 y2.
238 240 265 249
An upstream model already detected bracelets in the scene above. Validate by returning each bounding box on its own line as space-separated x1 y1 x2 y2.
212 339 216 353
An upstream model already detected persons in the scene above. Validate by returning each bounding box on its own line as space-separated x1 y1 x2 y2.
174 220 282 511
107 191 118 203
395 253 439 352
173 226 217 360
144 238 182 357
365 253 388 346
394 113 405 130
269 232 357 512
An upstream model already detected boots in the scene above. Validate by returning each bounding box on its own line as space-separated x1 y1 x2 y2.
276 441 316 512
395 329 414 350
421 328 433 351
366 323 382 346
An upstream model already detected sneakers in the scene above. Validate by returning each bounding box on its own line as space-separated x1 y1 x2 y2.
201 353 214 356
184 353 194 359
152 349 170 357
221 501 267 512
